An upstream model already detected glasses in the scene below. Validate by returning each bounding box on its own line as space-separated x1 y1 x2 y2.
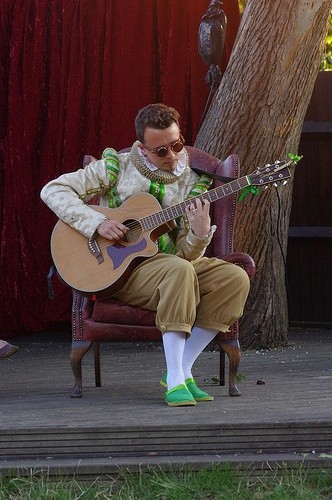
141 132 185 158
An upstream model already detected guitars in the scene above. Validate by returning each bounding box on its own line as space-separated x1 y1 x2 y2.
50 153 304 300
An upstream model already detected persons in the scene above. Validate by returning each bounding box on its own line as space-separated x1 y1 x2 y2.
39 103 250 407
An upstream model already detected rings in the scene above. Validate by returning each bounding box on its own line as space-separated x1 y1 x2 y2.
191 208 198 214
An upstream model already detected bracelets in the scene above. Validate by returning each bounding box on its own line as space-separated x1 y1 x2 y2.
97 217 107 228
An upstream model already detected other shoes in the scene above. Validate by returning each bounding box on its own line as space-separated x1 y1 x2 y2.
164 384 196 406
160 373 215 401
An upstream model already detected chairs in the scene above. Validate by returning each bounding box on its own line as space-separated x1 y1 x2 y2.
68 145 257 398
